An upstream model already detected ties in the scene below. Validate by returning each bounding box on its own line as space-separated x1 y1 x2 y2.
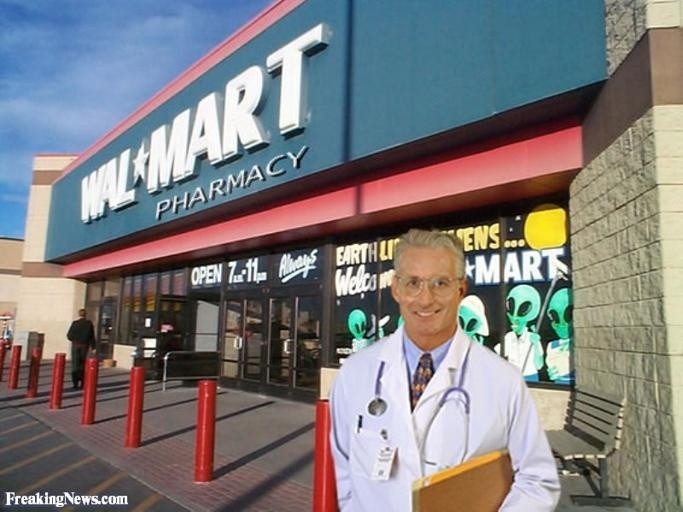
410 353 436 412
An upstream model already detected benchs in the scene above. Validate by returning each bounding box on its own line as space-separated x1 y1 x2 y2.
543 384 636 507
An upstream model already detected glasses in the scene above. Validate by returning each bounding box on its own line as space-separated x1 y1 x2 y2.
393 274 463 298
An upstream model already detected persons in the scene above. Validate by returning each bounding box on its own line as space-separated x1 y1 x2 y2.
327 227 562 512
66 309 97 390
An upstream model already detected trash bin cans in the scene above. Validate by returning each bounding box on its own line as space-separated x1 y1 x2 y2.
27 332 45 360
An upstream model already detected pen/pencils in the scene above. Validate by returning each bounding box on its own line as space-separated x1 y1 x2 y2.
358 415 362 434
